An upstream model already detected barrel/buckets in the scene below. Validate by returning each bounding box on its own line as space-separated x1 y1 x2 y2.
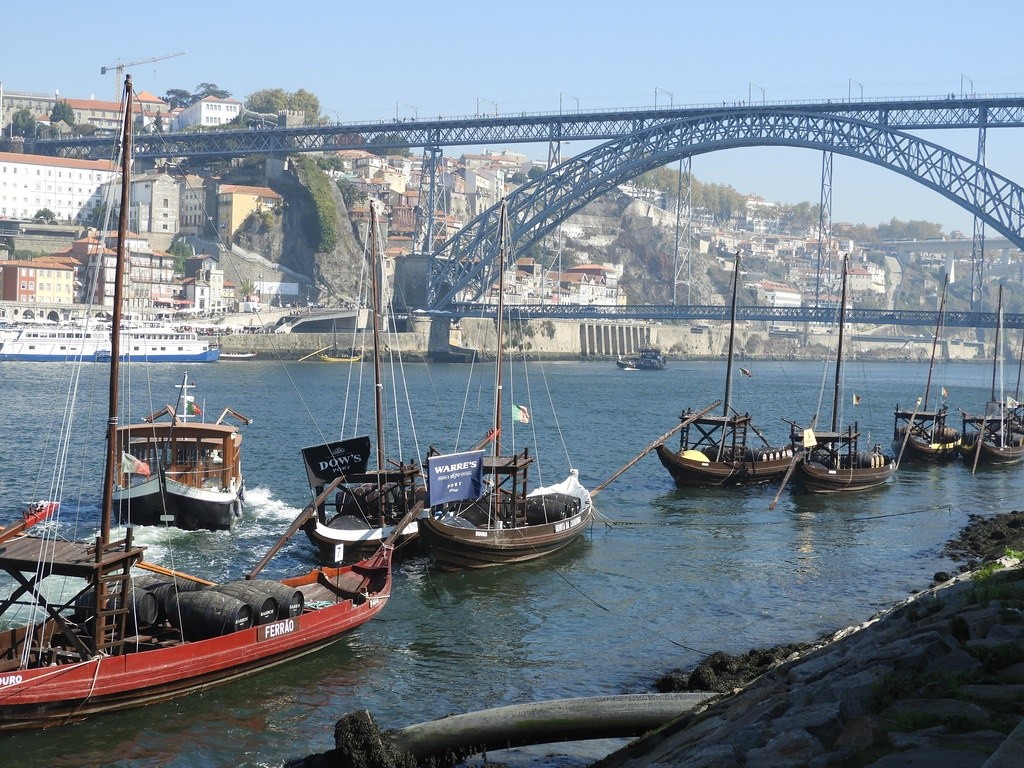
746 446 794 461
169 592 253 636
234 579 304 620
151 573 207 593
931 428 962 442
335 482 399 515
199 584 278 625
75 585 158 625
494 521 503 529
898 425 925 435
130 576 182 622
854 450 884 468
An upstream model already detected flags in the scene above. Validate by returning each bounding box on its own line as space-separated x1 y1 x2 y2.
853 394 861 405
941 386 949 398
511 405 530 424
739 368 751 377
187 401 202 415
121 451 150 477
1006 396 1019 408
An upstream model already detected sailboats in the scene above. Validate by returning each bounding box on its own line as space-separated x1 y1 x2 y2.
891 273 962 457
654 249 817 489
962 283 1024 464
791 250 898 492
0 73 594 717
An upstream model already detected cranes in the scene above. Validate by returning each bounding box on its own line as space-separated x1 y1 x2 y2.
102 48 187 119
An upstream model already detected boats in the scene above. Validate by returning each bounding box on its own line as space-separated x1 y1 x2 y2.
219 350 258 360
0 304 223 362
317 354 363 362
616 336 666 370
